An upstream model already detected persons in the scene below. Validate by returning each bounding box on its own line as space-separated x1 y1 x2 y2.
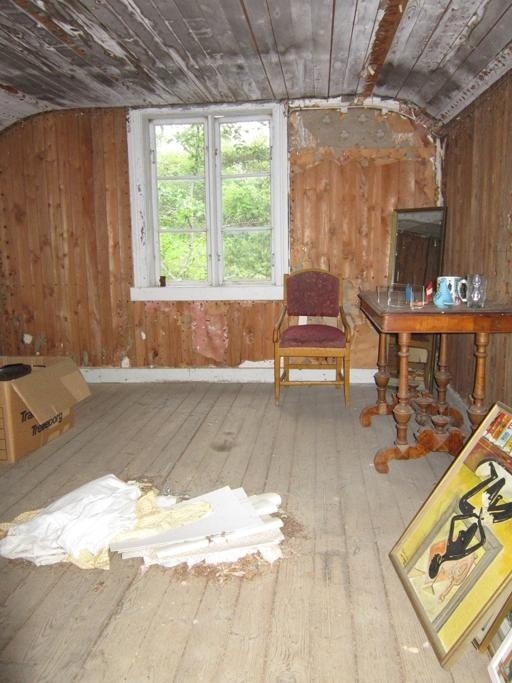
429 461 512 579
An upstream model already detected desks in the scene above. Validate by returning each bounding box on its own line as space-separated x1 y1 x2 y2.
358 288 512 471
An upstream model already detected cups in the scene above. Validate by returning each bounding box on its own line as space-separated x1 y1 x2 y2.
388 282 425 310
458 273 487 309
437 277 462 306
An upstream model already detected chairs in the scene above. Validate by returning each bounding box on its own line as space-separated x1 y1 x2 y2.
272 270 354 406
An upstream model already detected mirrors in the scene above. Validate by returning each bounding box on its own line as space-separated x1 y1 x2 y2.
385 205 448 393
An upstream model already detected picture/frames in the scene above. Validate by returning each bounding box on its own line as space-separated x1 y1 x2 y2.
389 398 512 682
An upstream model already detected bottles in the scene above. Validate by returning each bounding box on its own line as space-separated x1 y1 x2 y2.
432 279 454 309
